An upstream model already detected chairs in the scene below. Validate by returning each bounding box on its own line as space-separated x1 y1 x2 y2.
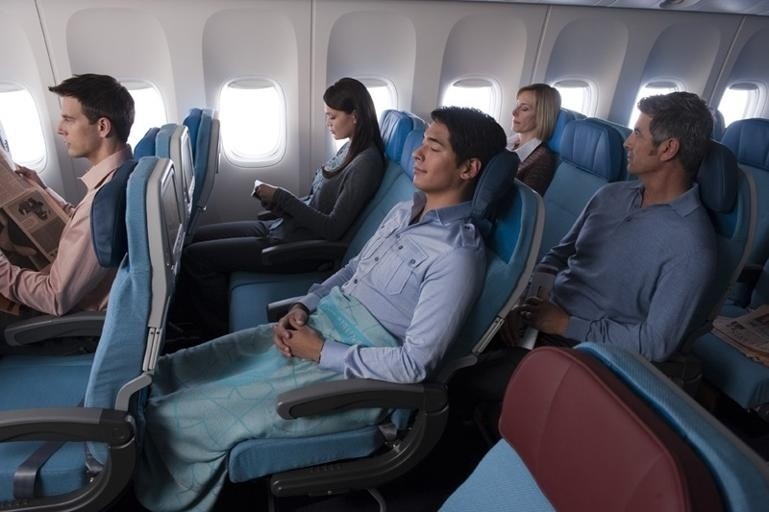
433 300 768 510
0 109 223 510
711 103 769 317
506 105 755 437
226 107 547 512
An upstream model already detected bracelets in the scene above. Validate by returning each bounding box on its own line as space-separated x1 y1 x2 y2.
319 341 324 363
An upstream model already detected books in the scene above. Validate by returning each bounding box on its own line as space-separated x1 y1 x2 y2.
249 180 294 221
510 271 556 350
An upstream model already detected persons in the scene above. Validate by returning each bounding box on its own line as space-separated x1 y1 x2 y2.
448 90 718 439
135 105 506 511
504 83 562 198
0 75 135 355
182 76 386 338
